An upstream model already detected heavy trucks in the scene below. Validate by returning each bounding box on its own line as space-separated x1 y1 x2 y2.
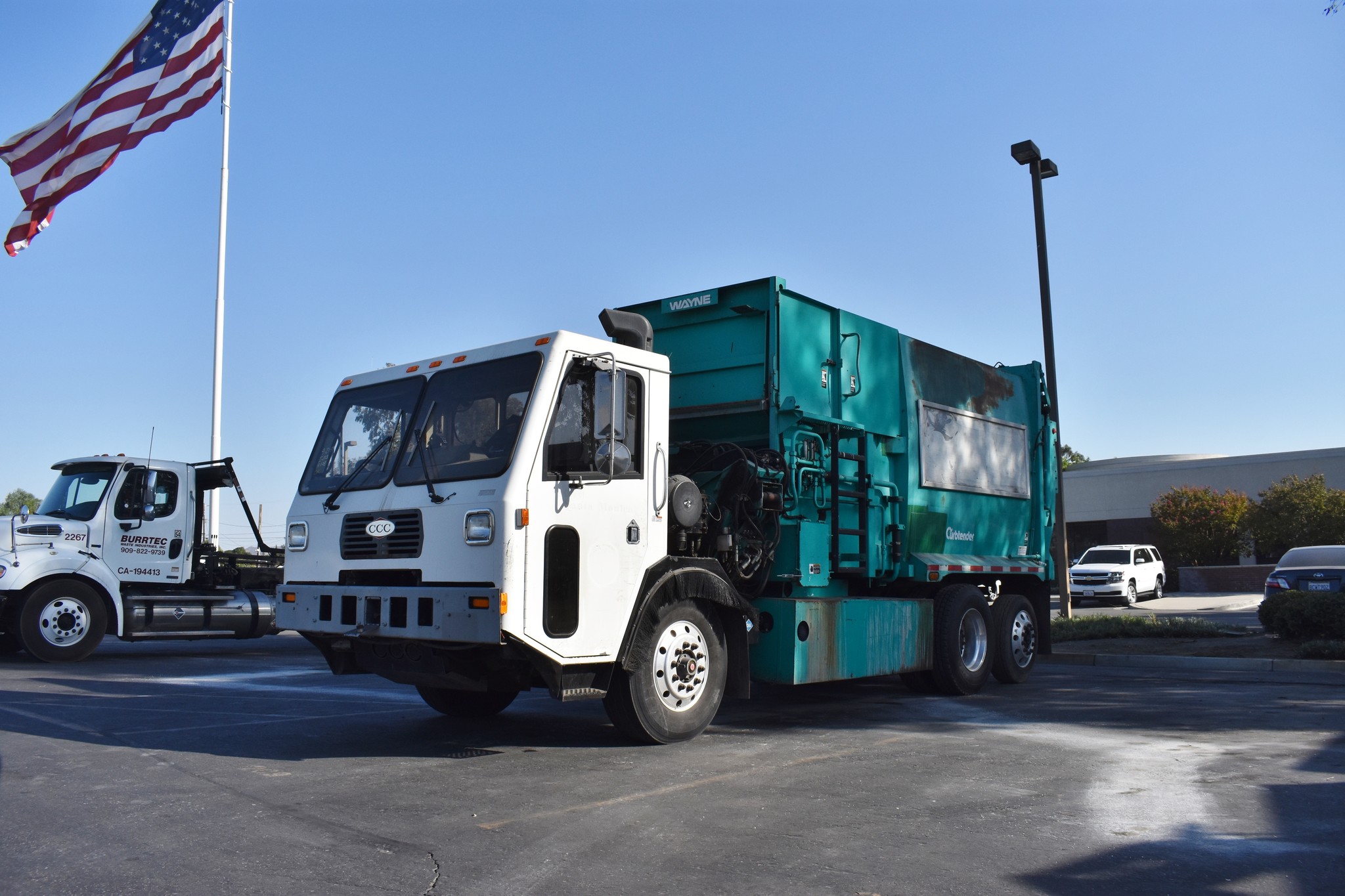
277 275 1060 743
0 451 286 666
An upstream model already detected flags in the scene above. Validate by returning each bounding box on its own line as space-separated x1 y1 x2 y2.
0 0 223 257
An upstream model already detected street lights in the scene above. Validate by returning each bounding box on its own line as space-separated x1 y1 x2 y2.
1009 138 1072 619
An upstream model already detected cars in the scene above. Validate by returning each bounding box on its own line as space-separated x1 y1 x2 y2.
1264 546 1345 602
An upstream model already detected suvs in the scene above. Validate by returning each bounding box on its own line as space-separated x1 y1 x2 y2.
1068 543 1166 607
